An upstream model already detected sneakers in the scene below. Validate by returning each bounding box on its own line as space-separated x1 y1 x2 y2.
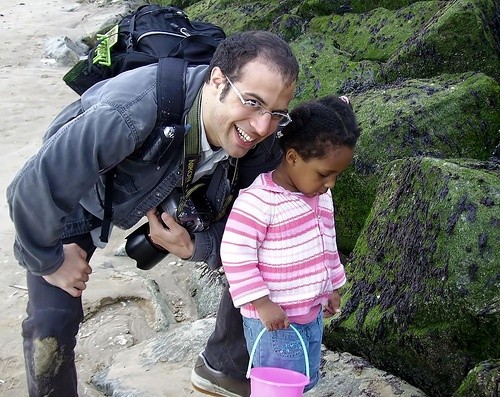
190 349 251 397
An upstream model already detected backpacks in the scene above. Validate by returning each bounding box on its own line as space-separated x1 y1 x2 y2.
62 4 260 243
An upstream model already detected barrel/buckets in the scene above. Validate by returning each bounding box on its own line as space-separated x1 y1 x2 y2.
246 323 310 397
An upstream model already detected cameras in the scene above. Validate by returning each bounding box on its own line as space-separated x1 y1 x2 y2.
125 187 217 270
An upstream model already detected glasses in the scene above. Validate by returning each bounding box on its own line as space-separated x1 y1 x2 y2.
225 74 292 126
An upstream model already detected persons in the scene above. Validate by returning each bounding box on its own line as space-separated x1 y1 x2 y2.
6 32 299 397
220 96 360 394
